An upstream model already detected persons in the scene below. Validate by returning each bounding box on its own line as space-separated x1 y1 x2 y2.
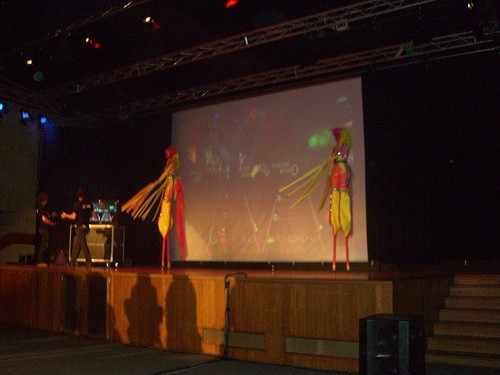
35 193 58 267
61 188 94 268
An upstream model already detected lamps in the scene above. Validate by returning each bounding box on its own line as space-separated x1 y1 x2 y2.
143 16 160 33
85 32 105 50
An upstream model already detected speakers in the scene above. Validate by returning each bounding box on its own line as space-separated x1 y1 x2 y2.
359 314 425 375
